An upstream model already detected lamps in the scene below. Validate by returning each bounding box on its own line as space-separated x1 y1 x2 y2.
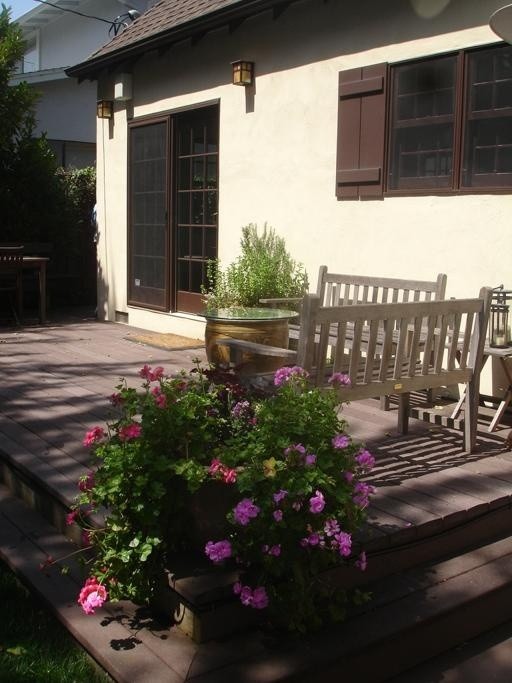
230 59 254 86
489 283 512 348
96 99 113 118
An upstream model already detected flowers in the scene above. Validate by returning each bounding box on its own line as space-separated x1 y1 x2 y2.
40 357 376 633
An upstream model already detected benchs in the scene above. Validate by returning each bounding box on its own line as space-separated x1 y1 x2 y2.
241 286 493 454
260 265 446 410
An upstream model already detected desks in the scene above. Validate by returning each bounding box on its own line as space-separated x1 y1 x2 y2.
0 257 51 323
444 338 512 432
198 307 299 369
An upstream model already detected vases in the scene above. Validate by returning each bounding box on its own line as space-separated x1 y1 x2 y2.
192 482 234 534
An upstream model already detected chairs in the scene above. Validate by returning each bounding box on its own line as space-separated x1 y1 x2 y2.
0 242 25 326
